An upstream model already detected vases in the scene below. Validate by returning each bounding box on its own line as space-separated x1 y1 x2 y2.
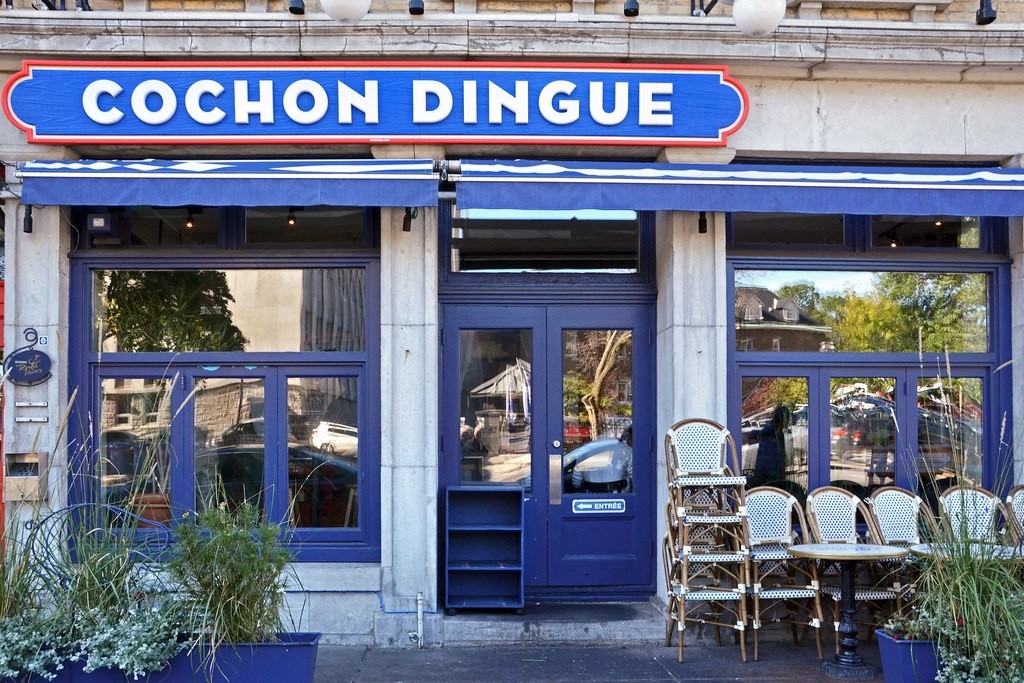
874 629 948 683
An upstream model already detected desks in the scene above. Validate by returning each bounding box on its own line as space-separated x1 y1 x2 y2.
909 542 1024 683
787 543 910 679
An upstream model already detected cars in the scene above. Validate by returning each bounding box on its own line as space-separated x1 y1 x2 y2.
98 381 983 540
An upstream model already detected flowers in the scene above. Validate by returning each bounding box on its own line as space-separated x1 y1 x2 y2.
884 608 966 640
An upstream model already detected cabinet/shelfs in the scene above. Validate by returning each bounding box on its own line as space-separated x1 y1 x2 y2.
444 485 525 609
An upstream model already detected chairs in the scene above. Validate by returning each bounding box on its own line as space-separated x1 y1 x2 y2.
661 418 754 664
735 485 1024 663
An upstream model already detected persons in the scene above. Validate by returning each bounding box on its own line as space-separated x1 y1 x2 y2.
458 414 528 479
572 427 634 493
753 405 790 484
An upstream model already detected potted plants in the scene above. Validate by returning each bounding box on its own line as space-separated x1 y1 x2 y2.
0 318 324 683
